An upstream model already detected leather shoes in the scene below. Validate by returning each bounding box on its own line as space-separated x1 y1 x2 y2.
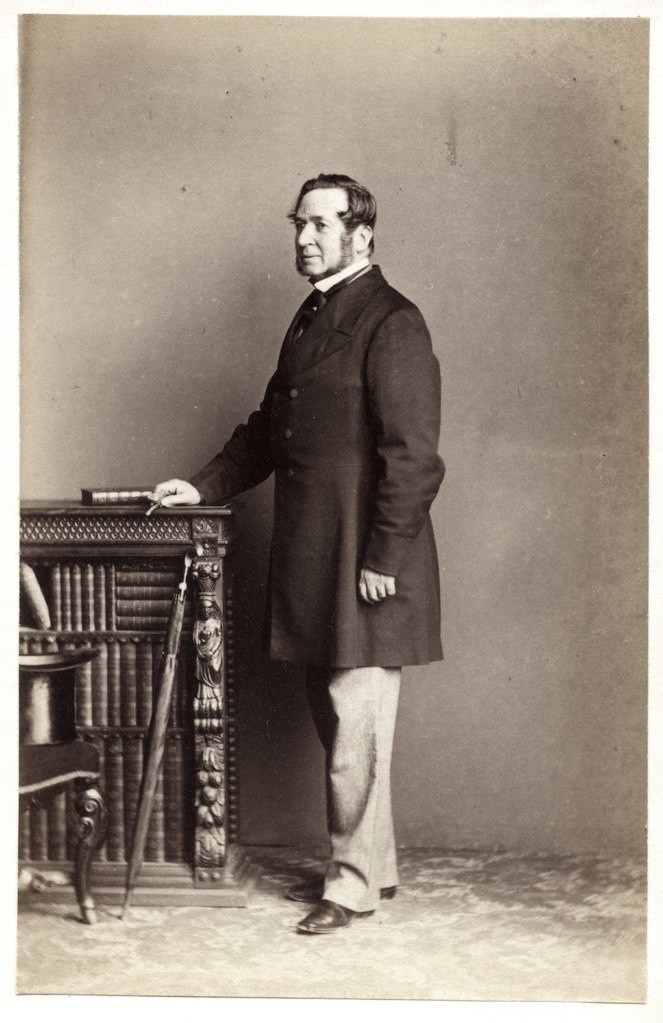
294 900 375 934
285 875 398 905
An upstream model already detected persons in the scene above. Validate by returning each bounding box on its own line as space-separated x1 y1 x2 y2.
147 167 448 936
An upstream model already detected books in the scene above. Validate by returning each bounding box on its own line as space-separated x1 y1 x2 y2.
19 561 193 635
20 640 196 729
20 739 196 866
79 486 158 505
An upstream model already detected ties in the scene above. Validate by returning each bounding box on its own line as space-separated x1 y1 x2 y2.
292 264 372 346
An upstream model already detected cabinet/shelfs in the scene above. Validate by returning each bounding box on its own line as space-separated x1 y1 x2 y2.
19 494 261 913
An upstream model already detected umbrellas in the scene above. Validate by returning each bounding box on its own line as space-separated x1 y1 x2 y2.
113 551 195 915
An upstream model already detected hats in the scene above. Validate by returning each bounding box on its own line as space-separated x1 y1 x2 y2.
19 645 104 746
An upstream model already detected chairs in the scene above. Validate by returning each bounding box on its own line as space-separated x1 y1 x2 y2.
19 646 109 923
